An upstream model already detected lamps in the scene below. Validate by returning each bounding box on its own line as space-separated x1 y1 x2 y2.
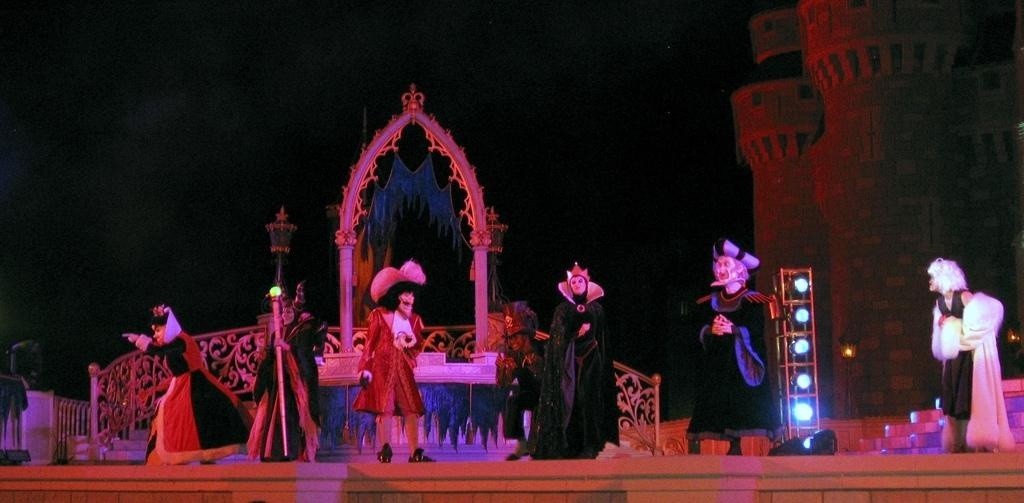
784 273 816 426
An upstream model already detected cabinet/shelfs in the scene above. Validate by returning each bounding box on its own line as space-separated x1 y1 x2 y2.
771 265 820 440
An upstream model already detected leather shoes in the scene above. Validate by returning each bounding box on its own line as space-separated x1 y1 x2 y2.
505 445 529 460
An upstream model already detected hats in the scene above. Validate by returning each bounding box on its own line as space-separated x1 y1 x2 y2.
566 261 591 286
502 301 539 338
712 239 760 273
370 258 426 305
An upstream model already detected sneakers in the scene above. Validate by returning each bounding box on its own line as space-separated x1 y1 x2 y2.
377 443 394 463
408 448 432 463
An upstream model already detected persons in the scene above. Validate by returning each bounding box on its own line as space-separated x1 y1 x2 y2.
246 306 327 464
926 258 1016 453
495 328 554 461
526 262 621 460
121 312 255 466
686 255 785 456
351 282 438 463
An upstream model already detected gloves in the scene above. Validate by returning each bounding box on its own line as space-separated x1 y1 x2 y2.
359 373 369 389
